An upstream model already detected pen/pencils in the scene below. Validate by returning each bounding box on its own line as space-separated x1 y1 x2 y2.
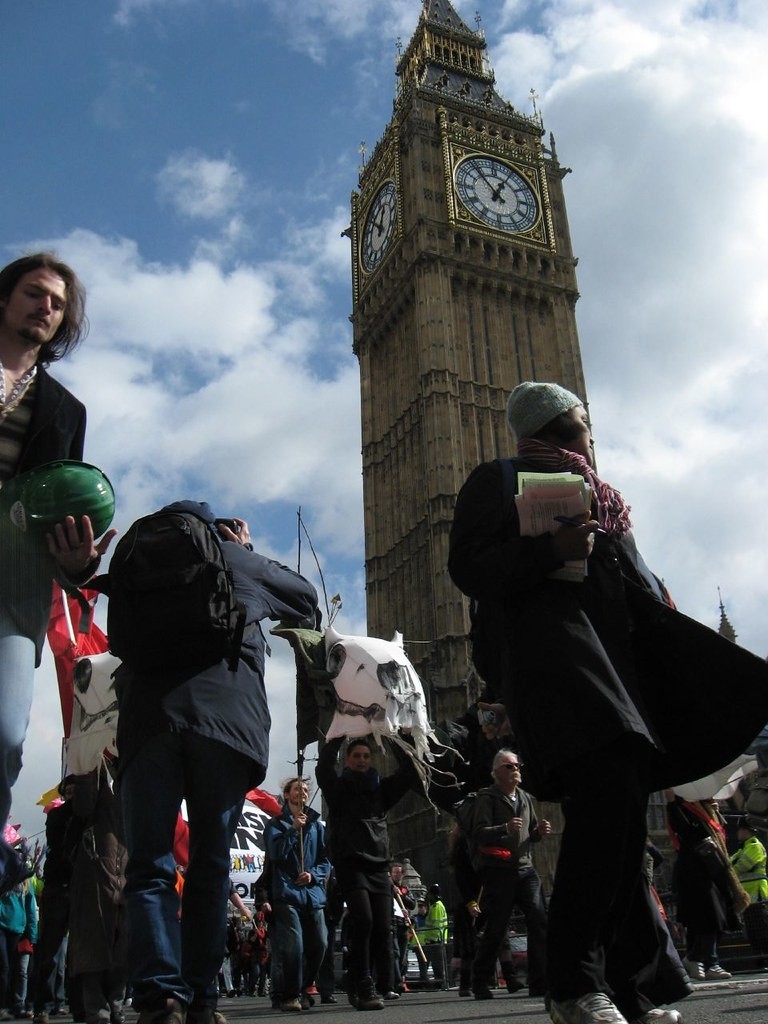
554 515 607 535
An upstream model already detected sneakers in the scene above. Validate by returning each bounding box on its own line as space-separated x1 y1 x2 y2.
706 964 732 979
545 992 629 1024
683 956 705 979
629 1007 683 1024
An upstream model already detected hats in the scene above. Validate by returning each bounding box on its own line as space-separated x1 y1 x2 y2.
506 382 585 441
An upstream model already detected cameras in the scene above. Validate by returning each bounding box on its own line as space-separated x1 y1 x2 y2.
214 517 239 542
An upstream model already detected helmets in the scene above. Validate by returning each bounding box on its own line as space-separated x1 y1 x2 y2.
735 815 758 832
429 884 441 896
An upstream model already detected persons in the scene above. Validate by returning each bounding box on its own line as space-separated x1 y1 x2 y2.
0 700 550 1024
0 253 119 834
645 788 768 981
103 500 318 1024
447 381 768 1024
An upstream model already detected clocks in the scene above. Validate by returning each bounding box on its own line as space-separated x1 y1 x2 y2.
356 181 406 273
455 153 542 234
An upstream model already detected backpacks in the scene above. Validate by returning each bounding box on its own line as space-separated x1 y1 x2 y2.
451 792 512 884
108 511 247 672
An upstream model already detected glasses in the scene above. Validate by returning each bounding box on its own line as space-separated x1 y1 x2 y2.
494 762 522 772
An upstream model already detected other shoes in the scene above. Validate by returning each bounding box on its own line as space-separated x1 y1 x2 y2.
0 980 410 1024
459 988 471 996
475 989 493 1000
507 981 524 993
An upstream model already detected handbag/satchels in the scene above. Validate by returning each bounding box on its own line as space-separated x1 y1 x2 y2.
675 836 724 878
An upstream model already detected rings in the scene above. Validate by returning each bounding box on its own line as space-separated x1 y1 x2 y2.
61 551 71 556
